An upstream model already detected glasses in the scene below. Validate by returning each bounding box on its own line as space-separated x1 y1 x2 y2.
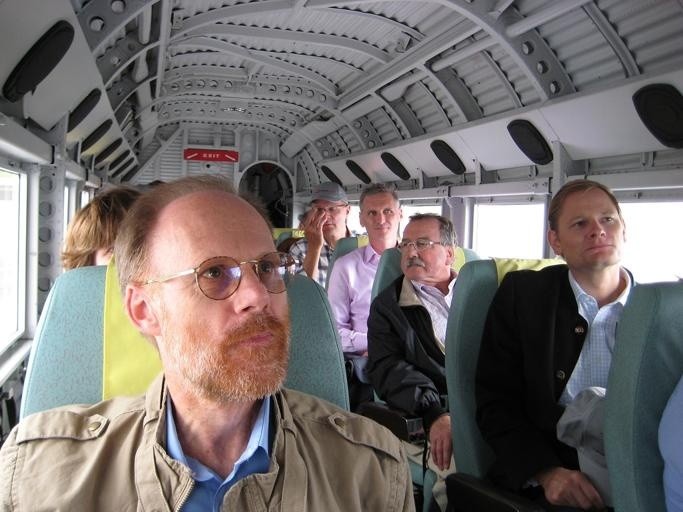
395 239 451 252
140 249 297 301
314 205 347 214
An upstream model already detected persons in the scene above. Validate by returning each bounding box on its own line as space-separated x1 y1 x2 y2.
473 177 642 511
57 178 145 271
284 181 361 290
324 183 403 414
364 212 459 512
0 169 418 512
276 211 310 253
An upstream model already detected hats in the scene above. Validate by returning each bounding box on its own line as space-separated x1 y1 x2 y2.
307 182 348 206
